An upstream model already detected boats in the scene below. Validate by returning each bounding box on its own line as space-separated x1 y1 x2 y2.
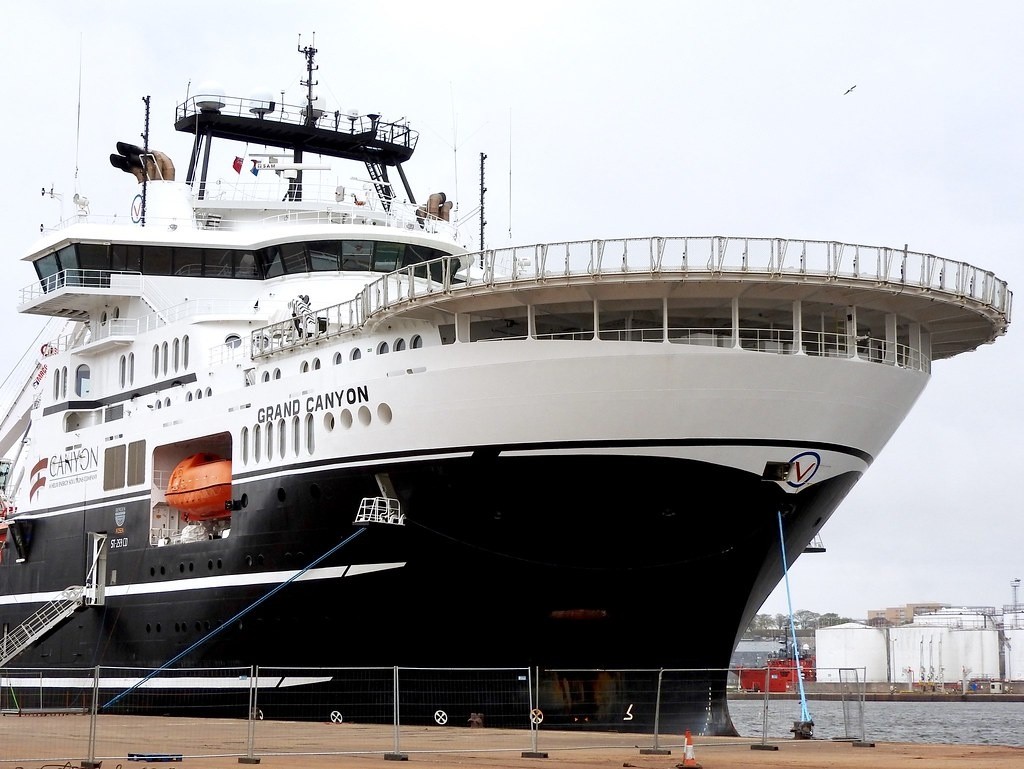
729 625 818 692
162 449 233 524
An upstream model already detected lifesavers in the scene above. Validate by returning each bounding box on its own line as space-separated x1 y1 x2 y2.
41 344 53 356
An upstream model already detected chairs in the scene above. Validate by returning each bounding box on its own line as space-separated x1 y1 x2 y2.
249 102 277 118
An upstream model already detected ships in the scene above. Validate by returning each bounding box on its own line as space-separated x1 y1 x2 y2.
1 27 1015 737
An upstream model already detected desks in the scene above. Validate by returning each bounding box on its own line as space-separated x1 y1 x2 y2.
196 101 226 114
300 109 326 127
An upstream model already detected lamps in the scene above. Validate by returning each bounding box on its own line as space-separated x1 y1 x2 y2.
73 194 89 215
